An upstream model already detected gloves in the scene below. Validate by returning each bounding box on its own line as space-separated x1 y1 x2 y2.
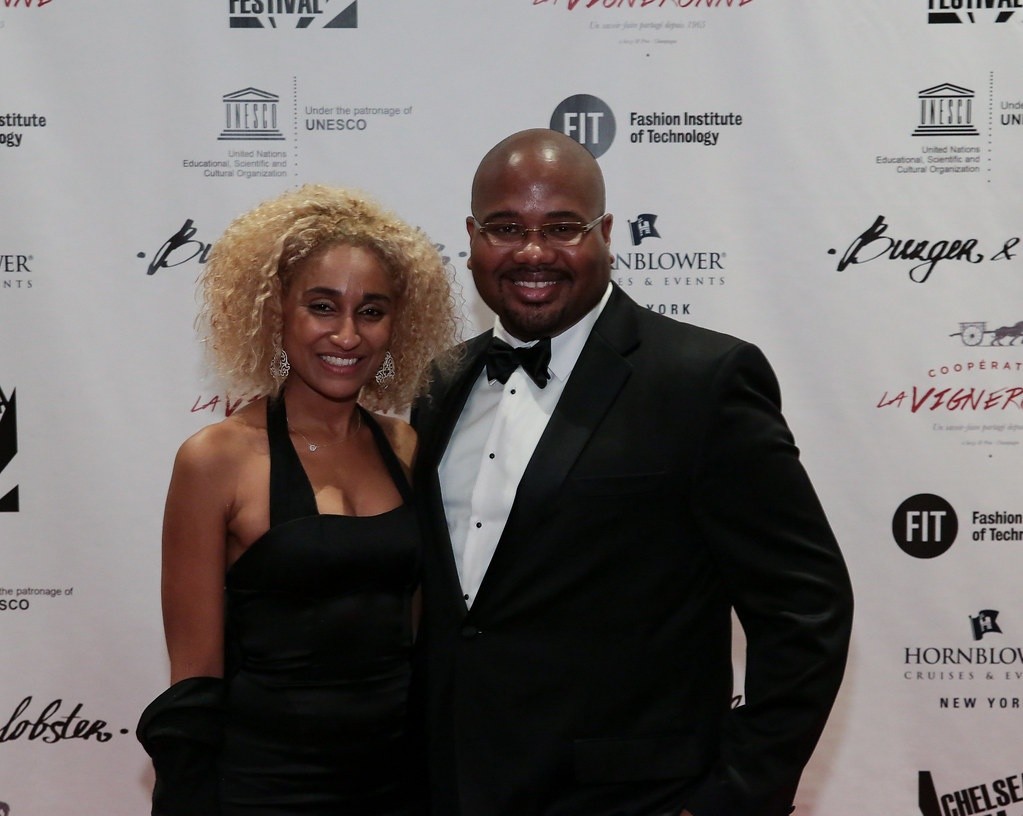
136 677 219 816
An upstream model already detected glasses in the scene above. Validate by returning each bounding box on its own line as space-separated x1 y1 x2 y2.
472 214 607 247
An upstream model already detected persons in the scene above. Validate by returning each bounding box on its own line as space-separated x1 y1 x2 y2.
136 184 452 816
408 128 854 816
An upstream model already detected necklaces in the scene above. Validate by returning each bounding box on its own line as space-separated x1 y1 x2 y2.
287 410 361 452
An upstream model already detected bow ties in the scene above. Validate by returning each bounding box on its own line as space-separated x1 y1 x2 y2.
483 336 552 389
